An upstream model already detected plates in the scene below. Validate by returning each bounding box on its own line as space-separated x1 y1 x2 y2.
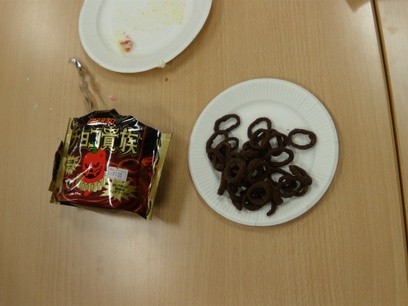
76 0 213 74
187 77 340 228
